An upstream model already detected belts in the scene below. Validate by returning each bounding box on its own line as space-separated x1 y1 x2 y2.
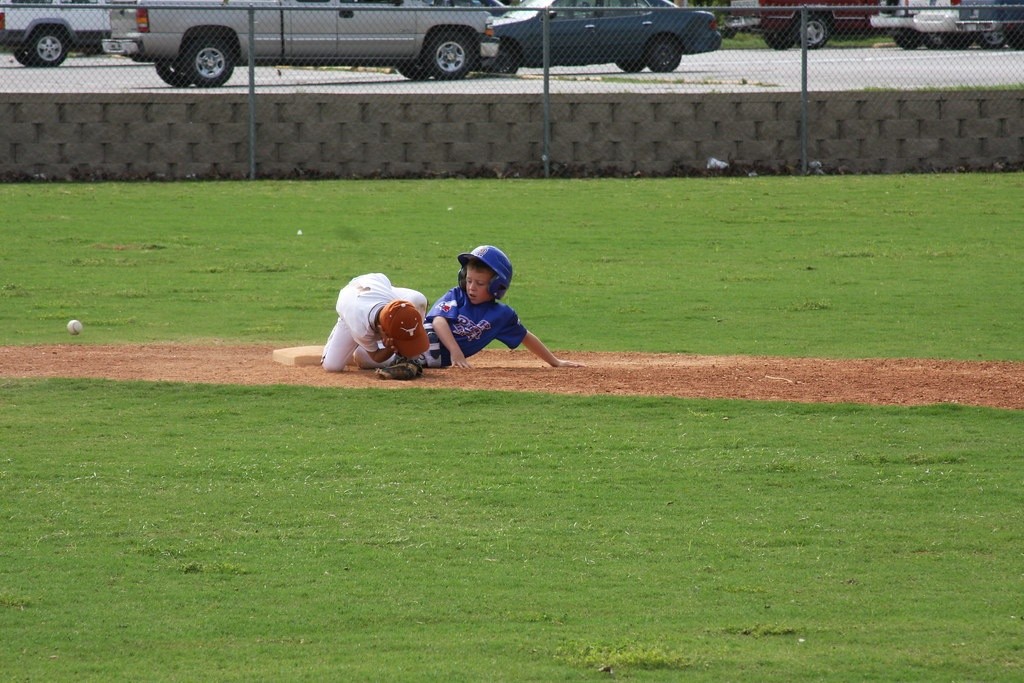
423 319 440 359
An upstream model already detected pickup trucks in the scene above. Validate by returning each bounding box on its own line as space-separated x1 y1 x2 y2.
0 0 131 69
99 1 495 88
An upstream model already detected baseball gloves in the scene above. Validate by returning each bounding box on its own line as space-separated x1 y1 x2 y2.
373 357 425 380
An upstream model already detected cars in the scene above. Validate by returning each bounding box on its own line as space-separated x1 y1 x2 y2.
490 0 721 76
673 0 1024 50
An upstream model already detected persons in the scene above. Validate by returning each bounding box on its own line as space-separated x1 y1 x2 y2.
353 245 587 381
321 273 429 370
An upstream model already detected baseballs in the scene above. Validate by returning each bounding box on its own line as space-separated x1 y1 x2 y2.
67 320 83 336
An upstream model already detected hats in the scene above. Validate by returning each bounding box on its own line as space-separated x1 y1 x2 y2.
379 300 430 356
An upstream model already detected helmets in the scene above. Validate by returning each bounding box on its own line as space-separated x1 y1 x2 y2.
457 245 513 299
